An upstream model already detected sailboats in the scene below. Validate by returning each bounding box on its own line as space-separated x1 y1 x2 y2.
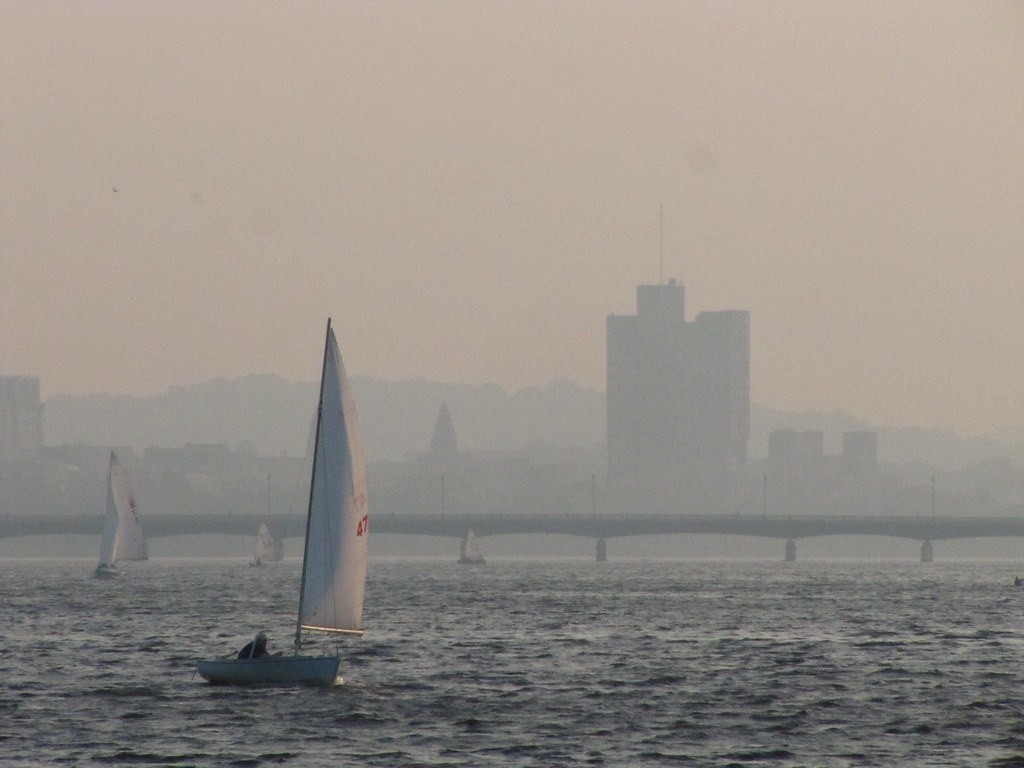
456 528 486 566
248 521 284 568
193 315 370 690
96 449 150 581
